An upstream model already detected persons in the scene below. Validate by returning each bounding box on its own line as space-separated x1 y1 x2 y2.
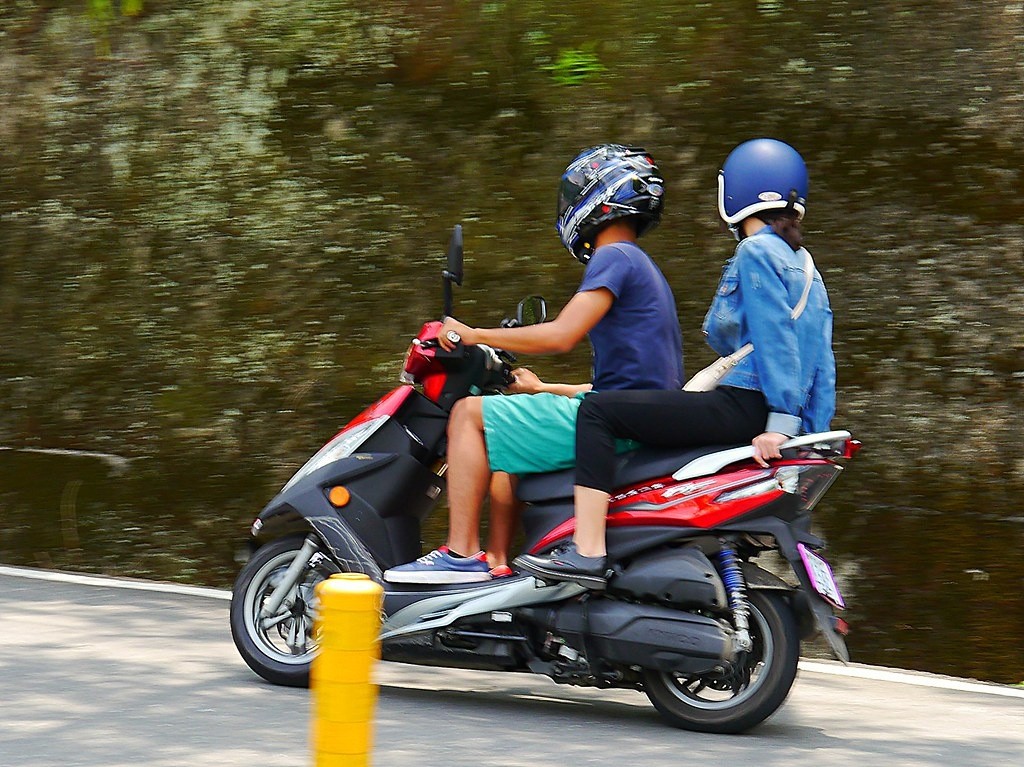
384 146 681 585
510 138 836 590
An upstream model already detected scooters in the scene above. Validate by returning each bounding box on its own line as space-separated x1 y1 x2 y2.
230 223 861 734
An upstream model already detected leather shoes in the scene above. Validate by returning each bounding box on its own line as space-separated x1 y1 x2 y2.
512 541 609 591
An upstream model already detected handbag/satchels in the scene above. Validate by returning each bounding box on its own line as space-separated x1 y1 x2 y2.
681 356 734 394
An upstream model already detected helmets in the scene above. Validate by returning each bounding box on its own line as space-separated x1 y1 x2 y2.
715 138 807 224
556 145 665 265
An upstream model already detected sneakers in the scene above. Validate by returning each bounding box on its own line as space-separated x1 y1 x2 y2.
383 545 492 584
